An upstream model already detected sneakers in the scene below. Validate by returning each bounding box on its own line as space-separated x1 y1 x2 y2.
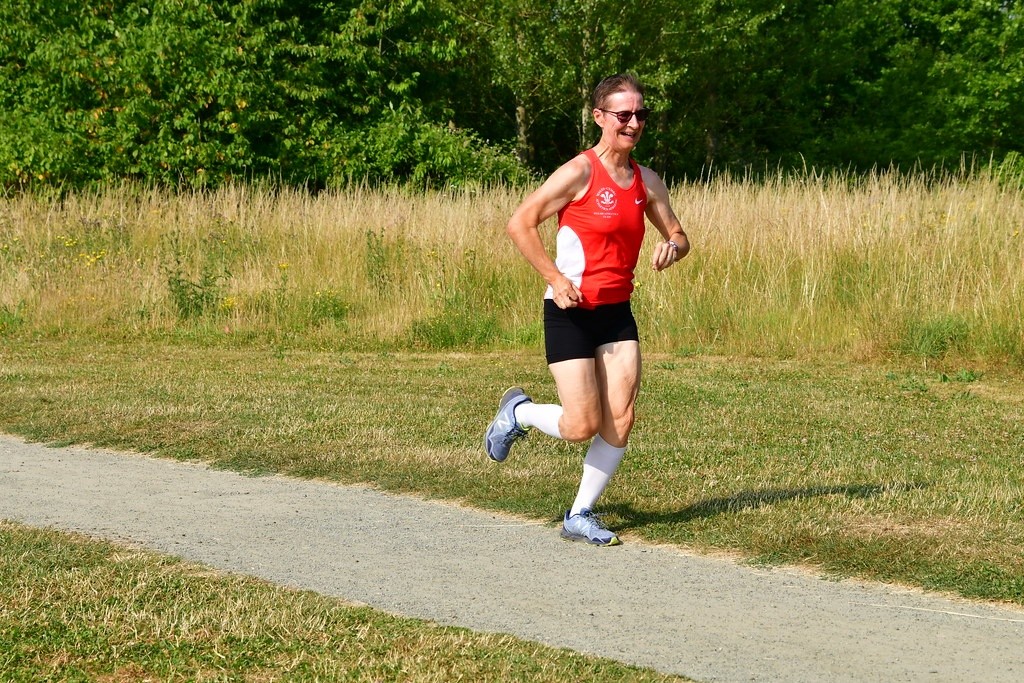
482 386 532 462
559 507 619 547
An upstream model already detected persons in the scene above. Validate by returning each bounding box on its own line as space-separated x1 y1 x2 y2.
484 74 690 546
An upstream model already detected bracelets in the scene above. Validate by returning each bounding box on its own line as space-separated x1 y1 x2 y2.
668 241 678 250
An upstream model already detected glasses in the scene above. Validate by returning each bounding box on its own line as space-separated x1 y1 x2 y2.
598 108 651 123
